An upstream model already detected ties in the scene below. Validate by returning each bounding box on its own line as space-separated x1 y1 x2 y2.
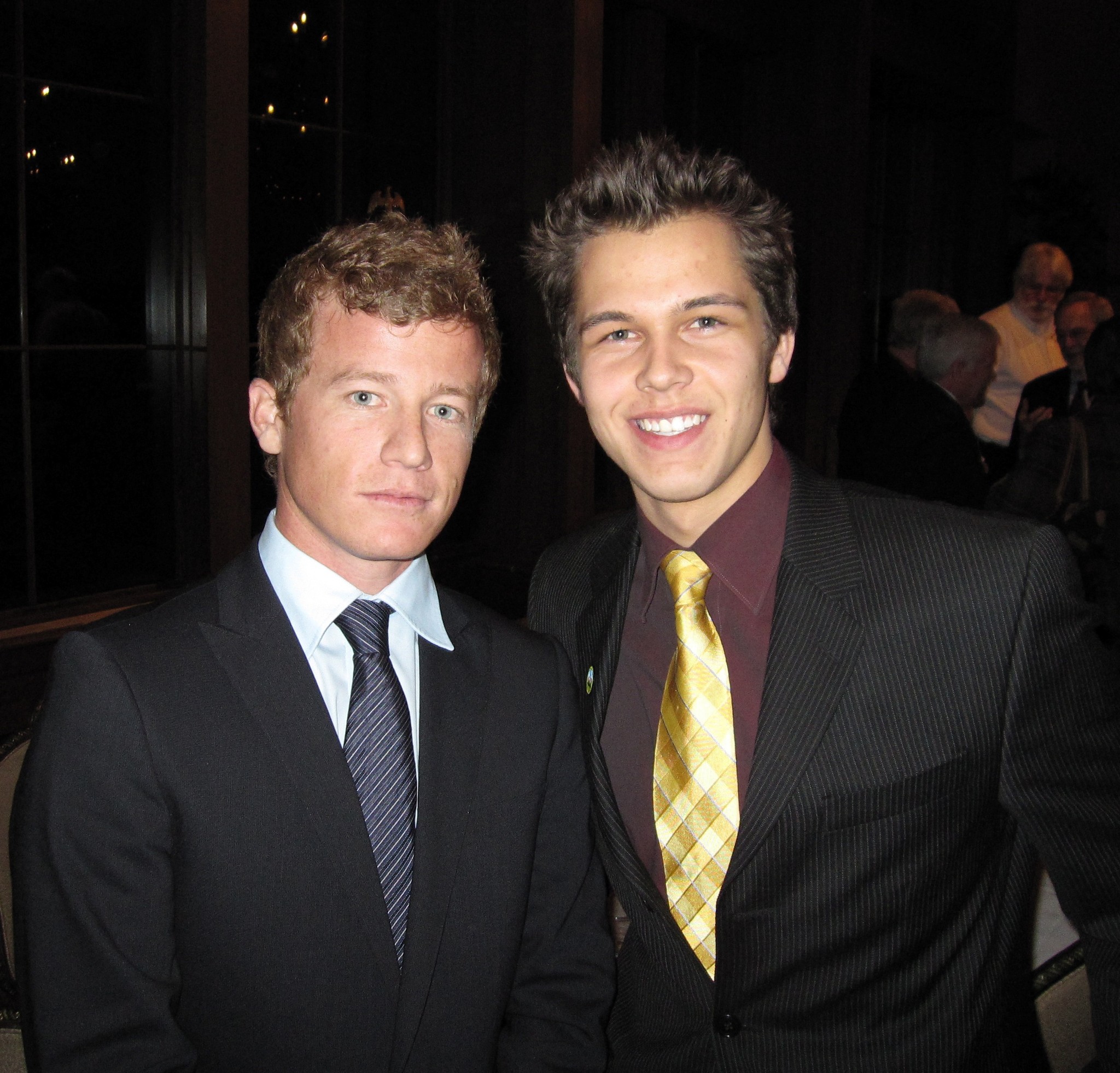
335 599 418 976
1053 378 1089 536
655 548 741 982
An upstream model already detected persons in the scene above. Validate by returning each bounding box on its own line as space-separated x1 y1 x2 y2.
512 133 1119 1073
0 216 637 1073
853 239 1119 650
